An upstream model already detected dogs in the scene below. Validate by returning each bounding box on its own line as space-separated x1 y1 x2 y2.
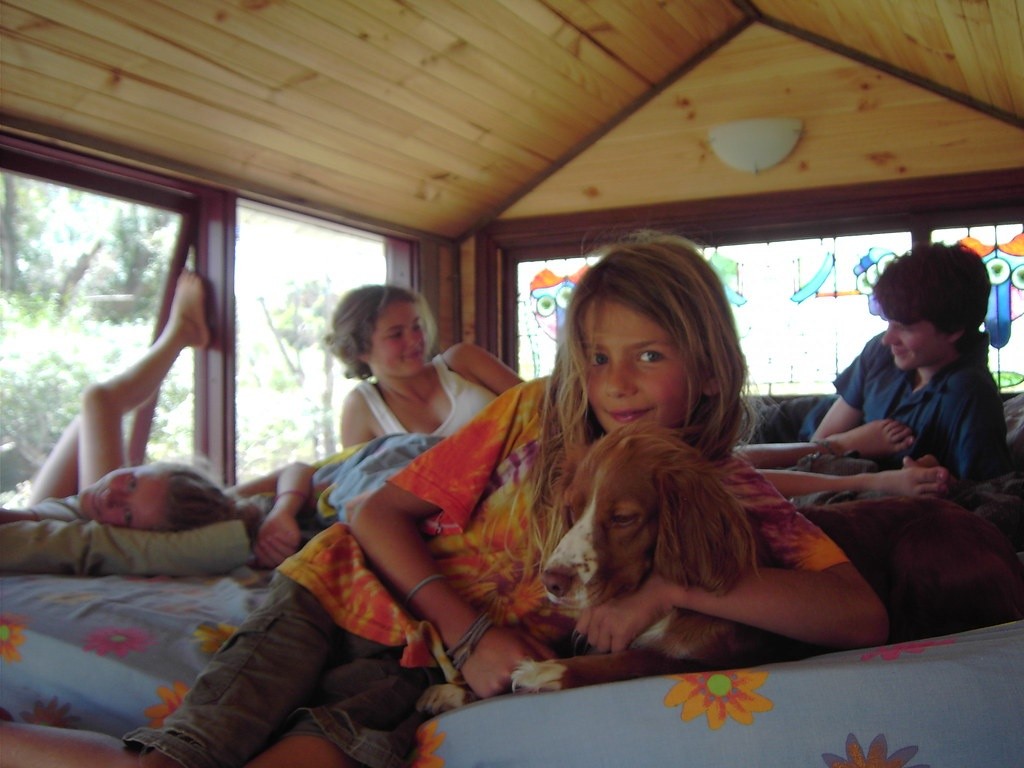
505 418 1024 699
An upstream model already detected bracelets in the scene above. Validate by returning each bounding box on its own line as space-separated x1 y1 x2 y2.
272 490 306 504
403 572 449 614
443 605 497 671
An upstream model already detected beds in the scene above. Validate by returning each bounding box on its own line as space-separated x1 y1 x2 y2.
0 568 1024 768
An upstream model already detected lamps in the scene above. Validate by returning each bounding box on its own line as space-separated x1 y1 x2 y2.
709 117 805 172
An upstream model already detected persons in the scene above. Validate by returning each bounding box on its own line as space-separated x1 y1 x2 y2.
0 267 315 572
322 284 951 501
0 228 891 768
740 241 1010 500
